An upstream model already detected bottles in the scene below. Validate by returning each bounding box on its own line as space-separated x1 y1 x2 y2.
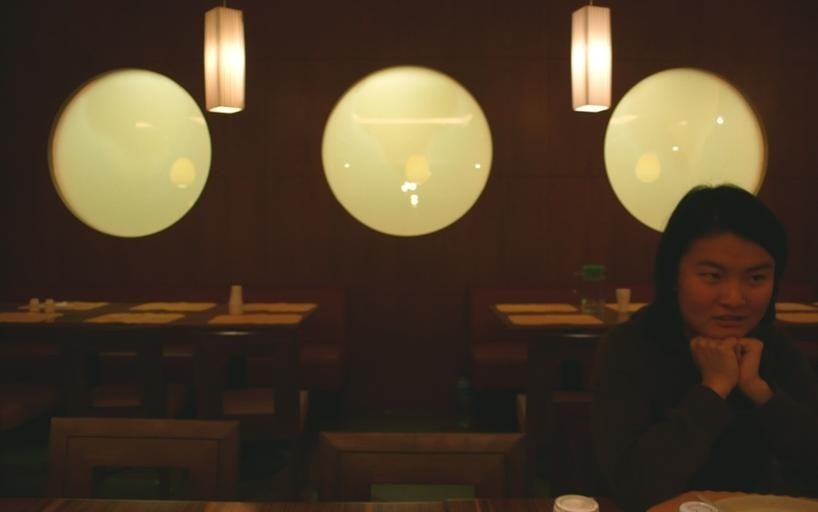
228 284 245 315
578 263 607 314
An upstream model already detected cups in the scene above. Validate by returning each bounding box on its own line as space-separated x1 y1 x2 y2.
678 499 716 512
551 493 601 512
29 297 41 313
45 299 54 313
615 288 632 316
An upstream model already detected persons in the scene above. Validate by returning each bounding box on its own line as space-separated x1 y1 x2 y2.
588 184 817 512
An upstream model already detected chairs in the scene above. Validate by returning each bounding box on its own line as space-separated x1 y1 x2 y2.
42 412 240 500
461 283 815 433
315 425 533 505
2 281 350 421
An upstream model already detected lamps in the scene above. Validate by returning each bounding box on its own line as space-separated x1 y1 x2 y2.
565 0 614 118
199 1 247 118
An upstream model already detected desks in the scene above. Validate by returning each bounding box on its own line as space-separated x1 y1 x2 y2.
0 492 641 510
